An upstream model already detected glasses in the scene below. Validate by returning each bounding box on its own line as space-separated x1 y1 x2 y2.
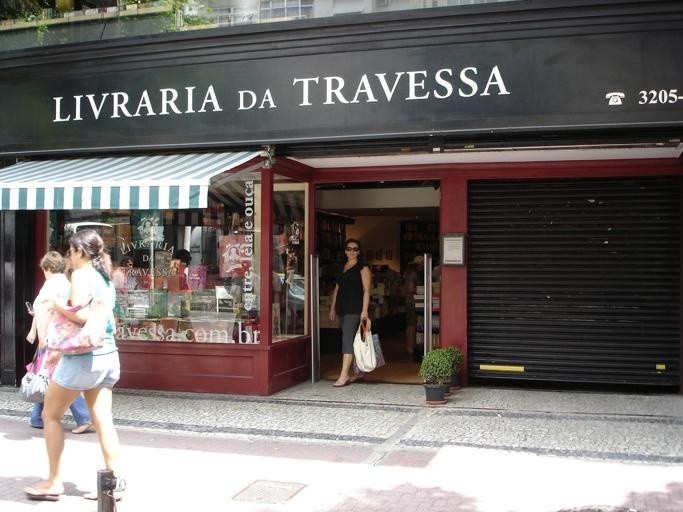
346 247 359 251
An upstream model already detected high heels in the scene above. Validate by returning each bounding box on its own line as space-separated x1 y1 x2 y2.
332 374 364 387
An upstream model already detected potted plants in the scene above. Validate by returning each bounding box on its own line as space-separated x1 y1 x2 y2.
419 347 462 402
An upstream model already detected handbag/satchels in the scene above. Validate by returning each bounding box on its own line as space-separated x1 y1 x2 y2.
352 318 385 373
18 370 48 402
46 307 105 356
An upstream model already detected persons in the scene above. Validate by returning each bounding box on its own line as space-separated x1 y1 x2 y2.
121 257 134 268
142 221 155 238
380 255 441 362
22 229 127 501
173 249 191 266
225 247 251 272
329 239 373 387
25 251 94 433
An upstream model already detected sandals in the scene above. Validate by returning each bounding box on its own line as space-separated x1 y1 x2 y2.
84 484 125 502
29 417 44 428
72 422 94 434
23 481 63 496
